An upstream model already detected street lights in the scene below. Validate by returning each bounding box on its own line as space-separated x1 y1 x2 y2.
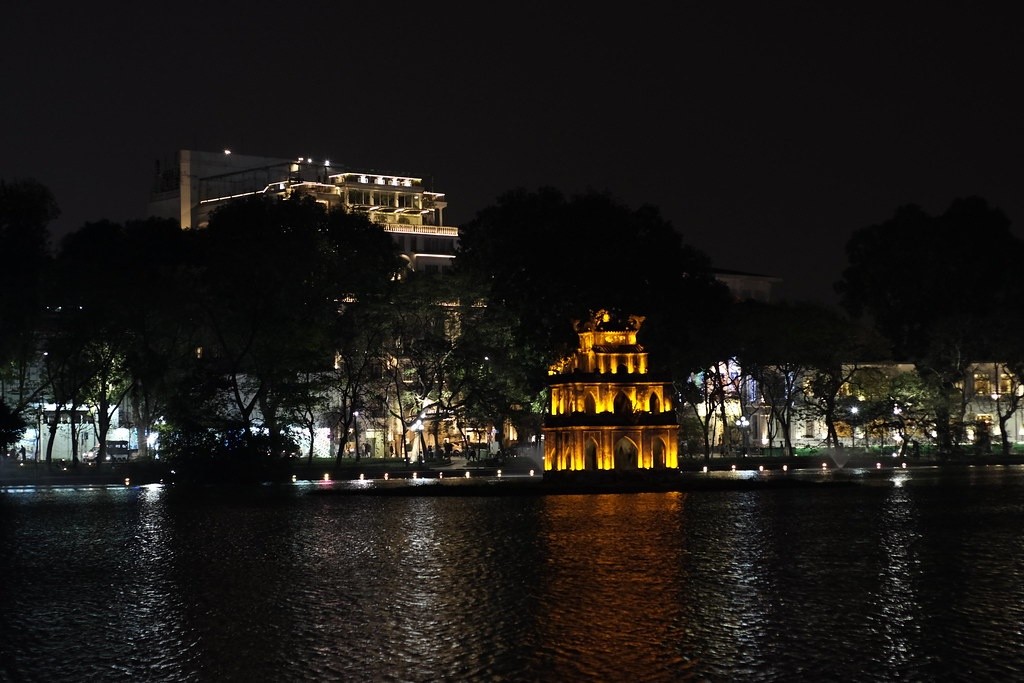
413 420 423 471
736 416 749 469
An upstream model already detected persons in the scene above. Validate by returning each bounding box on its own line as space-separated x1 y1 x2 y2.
361 439 477 463
19 445 26 461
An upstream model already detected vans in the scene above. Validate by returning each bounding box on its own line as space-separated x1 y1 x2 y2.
82 441 128 463
